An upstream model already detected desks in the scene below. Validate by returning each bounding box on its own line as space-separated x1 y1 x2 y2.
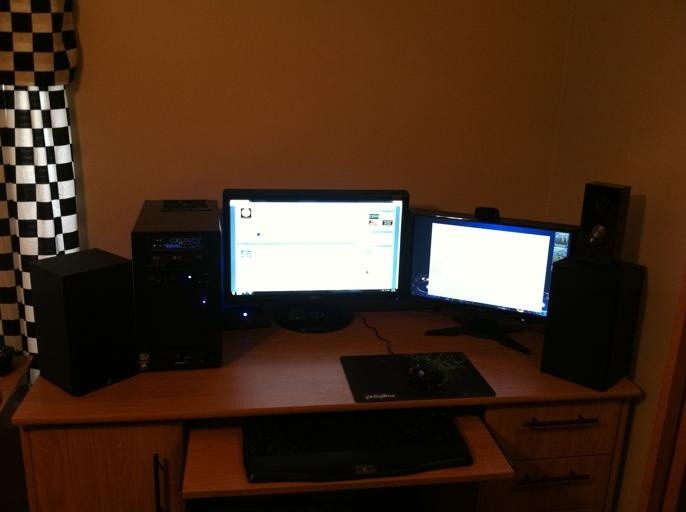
10 306 647 510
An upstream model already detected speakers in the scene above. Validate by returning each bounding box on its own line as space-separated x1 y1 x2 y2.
541 254 647 392
577 181 630 264
28 246 139 396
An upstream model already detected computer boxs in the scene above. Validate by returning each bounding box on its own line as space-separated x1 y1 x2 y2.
130 198 219 371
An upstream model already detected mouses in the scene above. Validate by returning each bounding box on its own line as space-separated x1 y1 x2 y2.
401 357 448 388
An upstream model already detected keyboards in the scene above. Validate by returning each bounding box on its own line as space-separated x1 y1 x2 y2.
242 408 473 483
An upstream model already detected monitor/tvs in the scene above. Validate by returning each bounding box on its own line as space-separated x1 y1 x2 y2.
407 209 582 355
219 188 410 333
